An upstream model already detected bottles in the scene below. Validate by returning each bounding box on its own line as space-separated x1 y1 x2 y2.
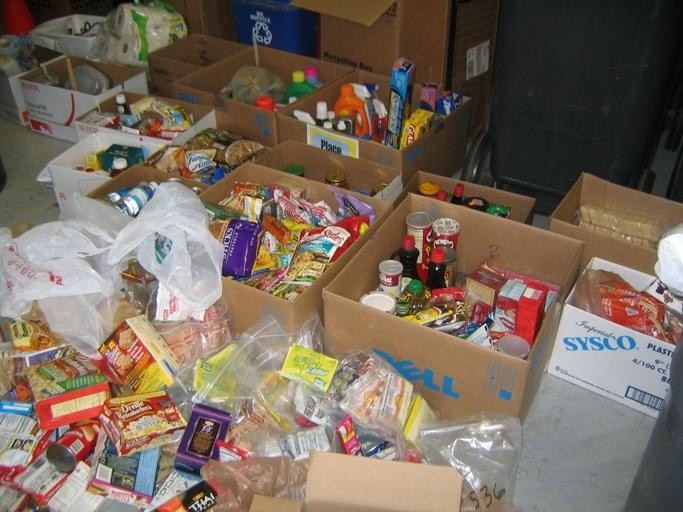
112 90 131 116
100 174 201 217
418 178 511 219
109 157 129 177
284 162 389 201
359 207 461 319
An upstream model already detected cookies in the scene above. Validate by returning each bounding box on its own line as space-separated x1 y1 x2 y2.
120 353 134 371
118 327 137 351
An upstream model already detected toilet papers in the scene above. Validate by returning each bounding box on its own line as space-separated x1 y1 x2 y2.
103 4 188 65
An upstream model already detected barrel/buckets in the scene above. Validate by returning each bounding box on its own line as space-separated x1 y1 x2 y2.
334 84 368 136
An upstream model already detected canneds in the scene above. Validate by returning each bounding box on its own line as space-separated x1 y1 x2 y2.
46 423 99 474
285 164 388 197
